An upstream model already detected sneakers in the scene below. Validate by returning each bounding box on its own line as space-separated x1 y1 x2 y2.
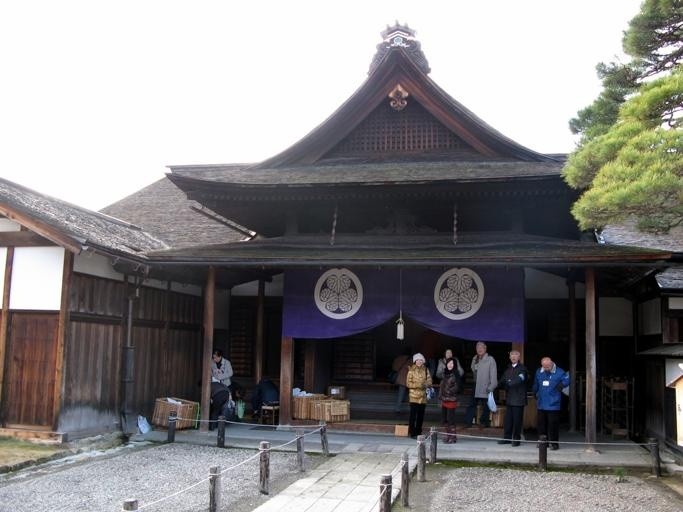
512 442 519 446
497 440 511 444
550 445 558 450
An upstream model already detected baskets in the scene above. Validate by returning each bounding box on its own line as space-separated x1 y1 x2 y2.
291 394 350 421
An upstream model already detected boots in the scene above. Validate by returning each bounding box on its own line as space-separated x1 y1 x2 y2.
444 422 456 444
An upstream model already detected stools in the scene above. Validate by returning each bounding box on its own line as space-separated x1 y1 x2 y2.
260 406 279 425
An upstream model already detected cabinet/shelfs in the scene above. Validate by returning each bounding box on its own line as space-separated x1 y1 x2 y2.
579 375 635 441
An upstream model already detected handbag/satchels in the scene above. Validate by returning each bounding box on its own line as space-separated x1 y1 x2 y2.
425 388 435 400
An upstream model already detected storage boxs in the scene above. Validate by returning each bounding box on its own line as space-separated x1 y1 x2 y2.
292 386 351 422
477 405 507 428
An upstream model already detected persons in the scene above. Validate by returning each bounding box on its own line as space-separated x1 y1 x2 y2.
405 353 432 439
209 382 229 430
437 358 458 446
464 341 497 430
211 351 233 390
249 378 278 421
530 356 571 452
434 349 465 381
393 353 412 419
496 347 527 447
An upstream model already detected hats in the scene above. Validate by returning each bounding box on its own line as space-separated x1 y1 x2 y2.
412 353 425 363
